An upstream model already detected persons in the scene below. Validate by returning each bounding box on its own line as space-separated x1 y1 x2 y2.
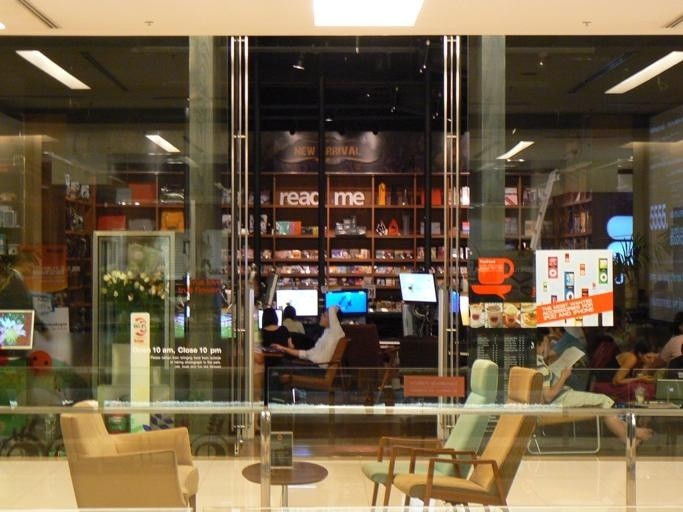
0 250 52 366
537 310 682 447
260 308 295 365
282 306 305 334
271 307 345 391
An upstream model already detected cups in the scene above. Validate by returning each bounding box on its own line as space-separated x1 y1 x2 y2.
486 307 501 327
469 306 482 328
475 256 514 284
635 388 646 403
401 212 410 235
505 312 517 326
377 177 386 204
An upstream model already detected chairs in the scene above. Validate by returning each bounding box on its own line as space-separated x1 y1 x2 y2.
361 358 500 509
267 339 356 443
58 400 199 512
394 365 546 510
528 407 600 457
354 324 437 436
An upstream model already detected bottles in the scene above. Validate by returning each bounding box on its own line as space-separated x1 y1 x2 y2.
388 214 399 235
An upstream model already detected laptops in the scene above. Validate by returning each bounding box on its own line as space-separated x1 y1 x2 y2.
654 378 683 401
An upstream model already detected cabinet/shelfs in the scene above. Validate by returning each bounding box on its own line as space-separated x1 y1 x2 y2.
42 152 593 314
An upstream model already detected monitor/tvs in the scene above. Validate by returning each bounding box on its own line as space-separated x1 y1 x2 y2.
258 308 284 330
275 289 318 319
324 288 369 317
450 291 462 314
398 272 438 305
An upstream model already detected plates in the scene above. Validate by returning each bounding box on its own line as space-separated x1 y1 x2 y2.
471 284 512 296
